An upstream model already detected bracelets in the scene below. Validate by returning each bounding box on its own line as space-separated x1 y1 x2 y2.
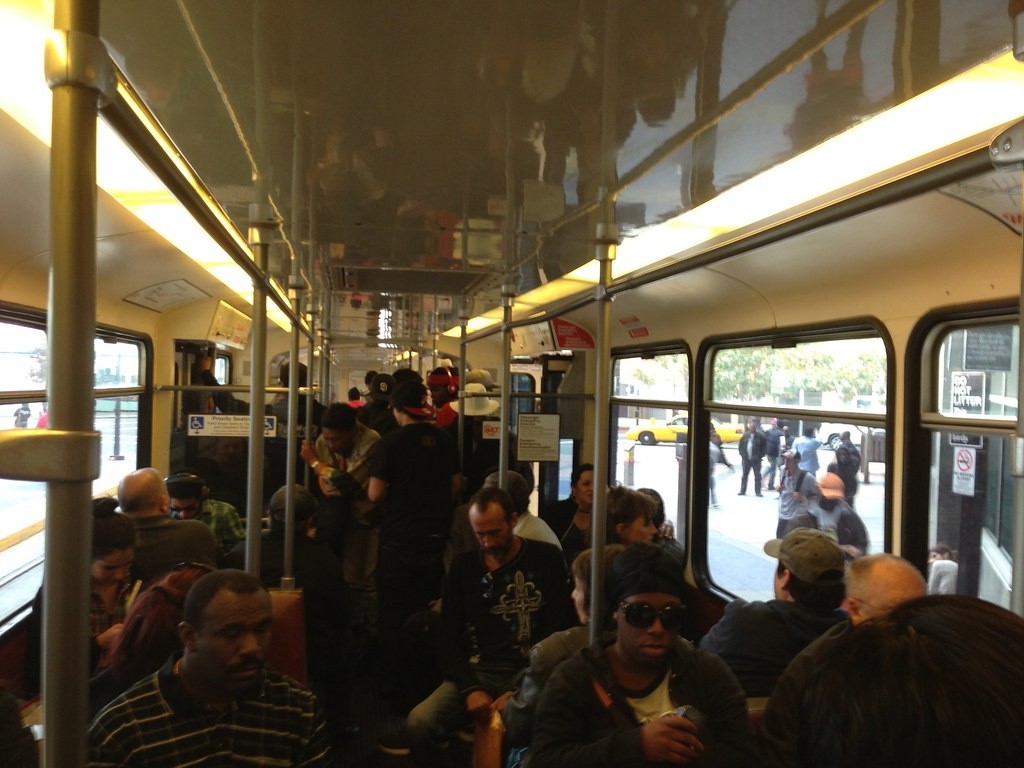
310 460 319 468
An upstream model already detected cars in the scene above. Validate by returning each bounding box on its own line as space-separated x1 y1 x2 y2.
819 422 885 451
625 412 745 447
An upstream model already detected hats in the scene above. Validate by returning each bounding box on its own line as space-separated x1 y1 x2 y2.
166 472 203 499
371 374 393 401
782 449 801 460
465 369 500 388
608 541 687 610
818 471 844 499
450 383 499 415
270 484 318 522
764 527 845 586
394 381 435 417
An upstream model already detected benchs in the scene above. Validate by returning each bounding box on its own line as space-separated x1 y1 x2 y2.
271 587 308 689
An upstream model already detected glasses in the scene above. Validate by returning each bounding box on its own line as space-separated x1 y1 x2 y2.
621 601 692 629
170 506 198 512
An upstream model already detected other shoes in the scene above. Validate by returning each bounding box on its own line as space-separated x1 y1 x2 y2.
340 721 366 733
379 736 450 754
756 493 764 497
739 492 746 495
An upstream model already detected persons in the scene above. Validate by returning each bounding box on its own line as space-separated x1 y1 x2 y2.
0 352 1024 768
